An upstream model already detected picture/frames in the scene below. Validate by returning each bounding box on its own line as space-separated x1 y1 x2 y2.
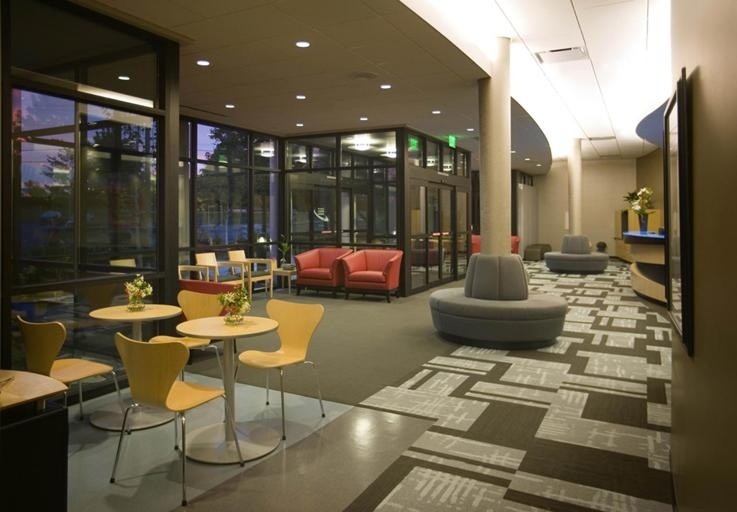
661 66 697 358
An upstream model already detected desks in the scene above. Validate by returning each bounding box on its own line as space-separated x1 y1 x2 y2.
89 304 183 432
0 369 68 512
622 231 669 309
175 315 281 465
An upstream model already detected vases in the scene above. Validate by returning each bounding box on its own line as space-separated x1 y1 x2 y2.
638 214 648 235
223 312 243 326
280 257 287 269
127 298 145 313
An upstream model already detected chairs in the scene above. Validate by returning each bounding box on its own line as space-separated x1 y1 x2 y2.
294 247 353 299
178 264 209 282
194 252 244 289
110 331 245 506
109 258 138 273
222 299 325 440
148 290 225 382
342 249 403 303
15 315 120 421
227 250 274 301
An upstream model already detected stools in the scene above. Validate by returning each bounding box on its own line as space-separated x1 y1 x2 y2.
273 267 297 297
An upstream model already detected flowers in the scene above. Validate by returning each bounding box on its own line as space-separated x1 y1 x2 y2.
623 183 657 214
218 286 250 325
277 233 291 258
123 273 153 312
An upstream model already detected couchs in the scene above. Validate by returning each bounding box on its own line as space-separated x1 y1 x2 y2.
429 253 568 350
543 234 608 275
410 241 446 266
